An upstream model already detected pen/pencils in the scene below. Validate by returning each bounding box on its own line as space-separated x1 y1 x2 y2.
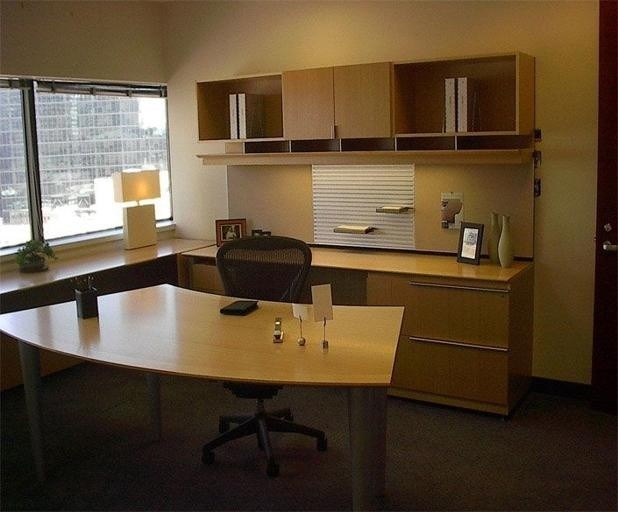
70 274 93 291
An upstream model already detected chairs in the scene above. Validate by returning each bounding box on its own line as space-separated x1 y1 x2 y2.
201 236 330 481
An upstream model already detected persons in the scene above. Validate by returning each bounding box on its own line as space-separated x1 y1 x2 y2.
224 227 237 241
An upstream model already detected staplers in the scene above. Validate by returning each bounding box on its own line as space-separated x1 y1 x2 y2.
273 317 284 343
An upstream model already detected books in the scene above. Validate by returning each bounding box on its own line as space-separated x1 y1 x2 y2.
237 93 265 139
219 300 258 316
229 93 240 139
456 77 481 133
444 78 458 133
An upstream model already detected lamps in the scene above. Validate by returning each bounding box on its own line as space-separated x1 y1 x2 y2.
112 168 162 252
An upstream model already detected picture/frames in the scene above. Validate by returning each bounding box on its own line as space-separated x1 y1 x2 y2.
214 217 248 247
456 220 485 266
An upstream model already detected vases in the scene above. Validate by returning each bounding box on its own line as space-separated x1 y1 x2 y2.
487 210 514 270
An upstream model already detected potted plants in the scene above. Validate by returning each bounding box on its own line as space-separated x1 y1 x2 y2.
14 236 58 275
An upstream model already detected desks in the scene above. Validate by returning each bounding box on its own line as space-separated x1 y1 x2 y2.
179 241 537 424
0 282 407 509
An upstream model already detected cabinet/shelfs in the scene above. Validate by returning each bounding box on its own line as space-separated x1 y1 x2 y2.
283 60 394 166
194 72 284 168
394 51 536 167
